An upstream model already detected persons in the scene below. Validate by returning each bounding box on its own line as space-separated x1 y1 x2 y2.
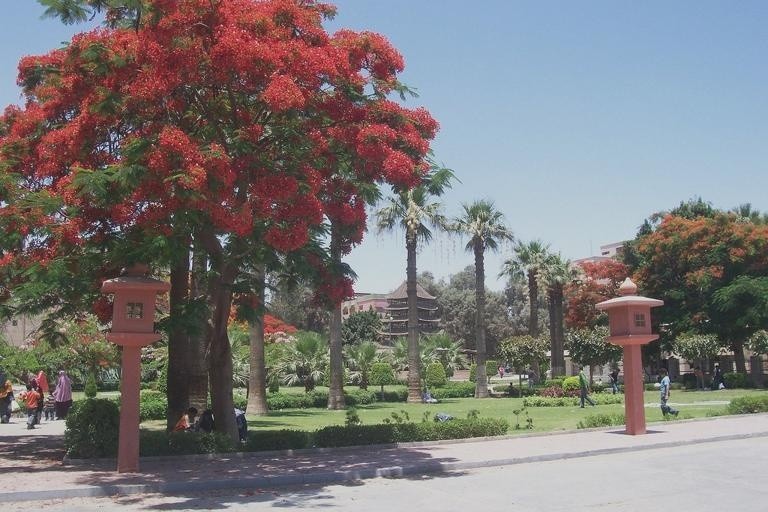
693 366 704 391
236 412 247 444
657 367 680 418
421 387 441 403
171 407 197 432
193 410 215 432
710 365 722 390
498 366 506 377
507 381 518 395
0 369 73 429
607 367 621 394
576 365 596 408
